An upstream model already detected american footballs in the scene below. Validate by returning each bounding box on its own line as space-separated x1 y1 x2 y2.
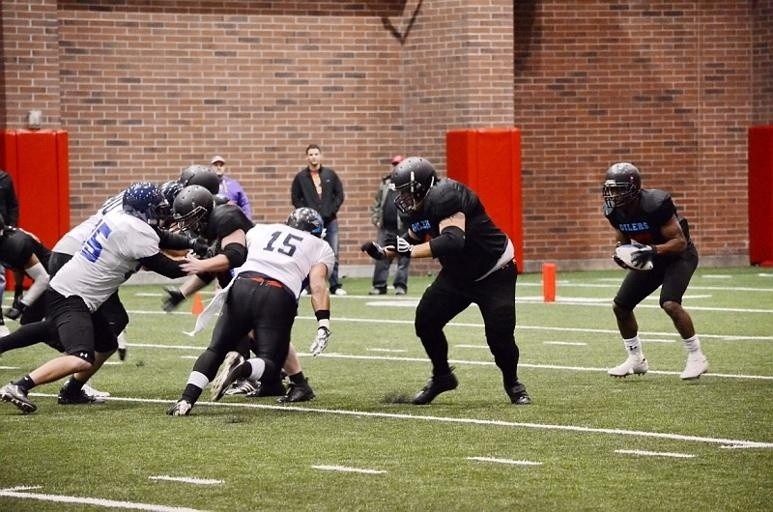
616 245 653 271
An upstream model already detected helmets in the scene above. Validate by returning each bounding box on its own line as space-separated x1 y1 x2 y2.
180 164 219 195
388 156 437 215
172 184 214 240
601 162 643 208
287 207 324 237
160 179 183 208
121 180 170 224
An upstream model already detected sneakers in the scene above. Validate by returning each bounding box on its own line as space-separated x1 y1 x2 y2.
59 391 104 406
72 380 109 398
163 399 190 416
1 383 36 413
410 369 460 405
393 286 405 296
606 356 648 379
369 289 382 296
335 289 347 297
506 380 532 406
679 354 709 381
206 352 315 405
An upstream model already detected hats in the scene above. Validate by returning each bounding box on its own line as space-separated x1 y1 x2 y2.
391 155 404 165
210 154 224 165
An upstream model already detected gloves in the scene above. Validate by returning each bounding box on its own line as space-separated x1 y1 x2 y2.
160 285 185 312
309 319 331 357
190 236 211 258
360 240 386 261
384 232 412 257
630 242 656 268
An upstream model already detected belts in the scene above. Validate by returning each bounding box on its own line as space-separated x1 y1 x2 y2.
247 275 282 288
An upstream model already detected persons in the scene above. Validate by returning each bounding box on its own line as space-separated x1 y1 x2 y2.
368 155 408 296
361 157 533 406
291 145 347 296
0 156 335 415
601 163 710 380
